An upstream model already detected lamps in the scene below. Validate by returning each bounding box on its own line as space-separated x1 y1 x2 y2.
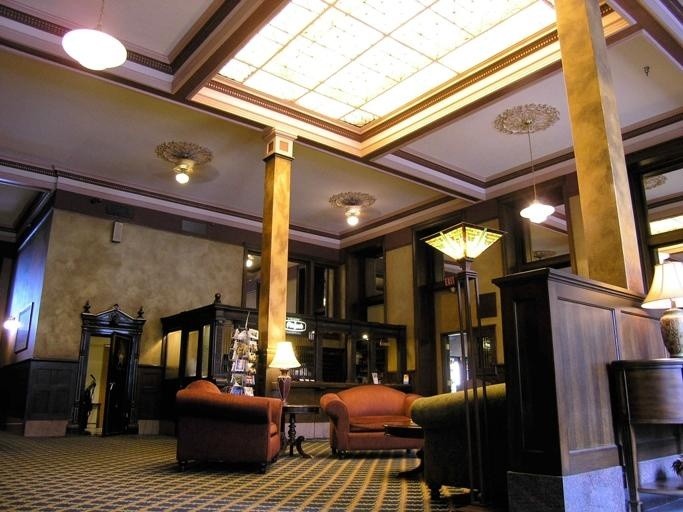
267 339 301 405
641 255 683 358
59 1 130 74
517 119 556 225
419 216 511 509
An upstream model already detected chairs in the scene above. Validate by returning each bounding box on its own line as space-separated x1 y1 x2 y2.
172 378 283 476
408 382 505 500
318 383 427 459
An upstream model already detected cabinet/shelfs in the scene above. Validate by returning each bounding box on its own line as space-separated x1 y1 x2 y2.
611 354 683 510
488 264 680 479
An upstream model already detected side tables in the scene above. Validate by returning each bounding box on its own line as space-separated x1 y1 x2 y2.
283 402 322 460
